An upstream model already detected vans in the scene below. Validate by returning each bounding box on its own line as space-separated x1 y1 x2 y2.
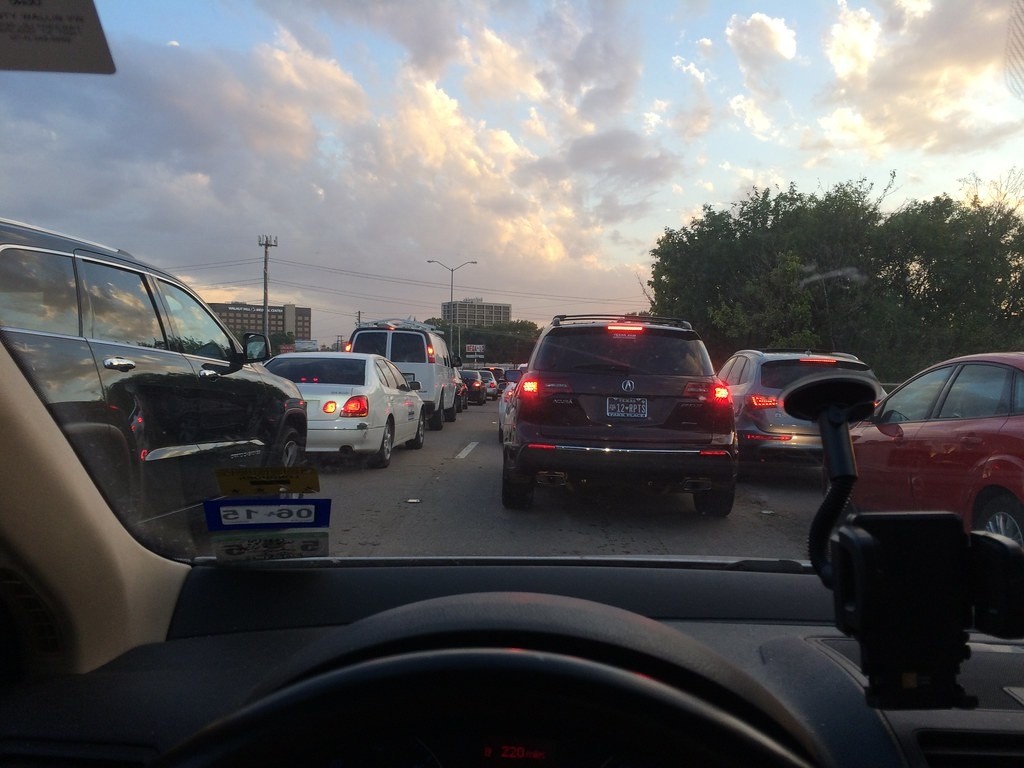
345 319 462 430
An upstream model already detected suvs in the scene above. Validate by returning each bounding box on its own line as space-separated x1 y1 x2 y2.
0 216 310 609
503 315 738 519
713 346 891 483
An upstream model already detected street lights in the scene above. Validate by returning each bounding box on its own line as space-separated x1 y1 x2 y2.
428 260 478 358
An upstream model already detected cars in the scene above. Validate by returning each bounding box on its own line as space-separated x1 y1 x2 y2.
498 365 534 443
262 351 426 469
458 367 508 406
823 350 1023 550
